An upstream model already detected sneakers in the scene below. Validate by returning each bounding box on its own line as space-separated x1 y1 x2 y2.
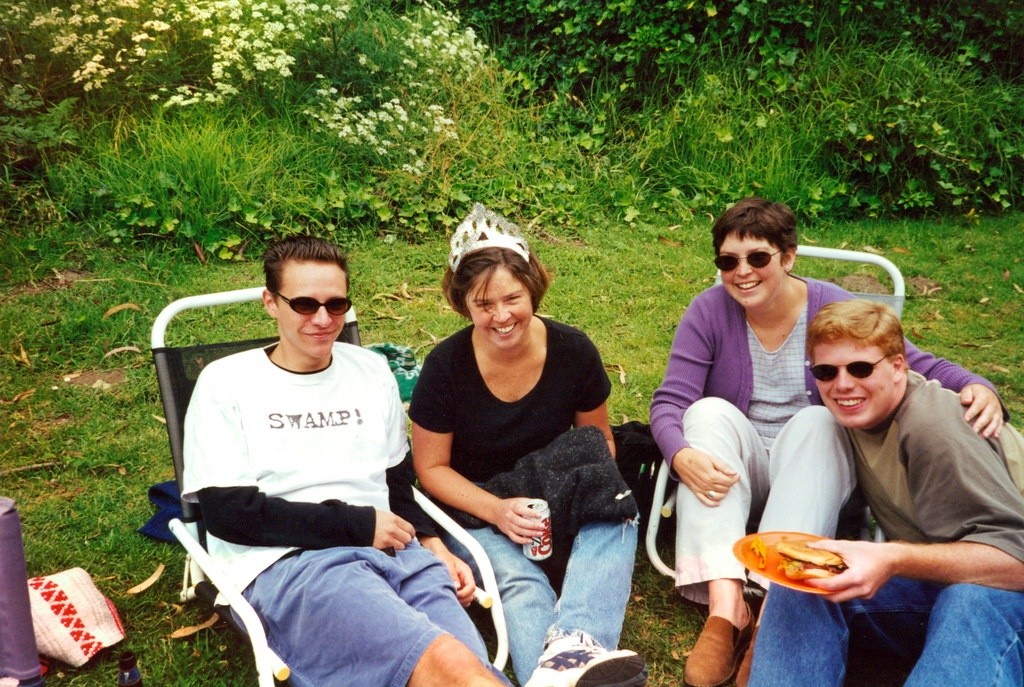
522 633 648 687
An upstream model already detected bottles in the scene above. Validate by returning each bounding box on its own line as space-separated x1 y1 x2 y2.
119 653 142 687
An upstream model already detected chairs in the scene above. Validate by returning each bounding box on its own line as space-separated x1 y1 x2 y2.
645 244 905 581
151 285 509 687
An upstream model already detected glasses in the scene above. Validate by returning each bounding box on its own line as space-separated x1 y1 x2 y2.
714 244 796 272
809 353 896 381
276 291 351 316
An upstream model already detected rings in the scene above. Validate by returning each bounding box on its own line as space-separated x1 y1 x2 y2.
707 491 715 499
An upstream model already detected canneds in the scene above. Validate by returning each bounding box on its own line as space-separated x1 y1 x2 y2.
522 499 552 561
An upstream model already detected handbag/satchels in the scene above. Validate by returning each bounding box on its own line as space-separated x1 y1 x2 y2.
609 422 680 542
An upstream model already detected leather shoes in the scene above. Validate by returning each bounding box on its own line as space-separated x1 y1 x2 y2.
685 601 759 687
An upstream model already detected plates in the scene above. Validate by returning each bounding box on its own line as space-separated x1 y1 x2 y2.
732 531 843 594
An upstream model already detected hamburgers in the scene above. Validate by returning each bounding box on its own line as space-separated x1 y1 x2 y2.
778 544 844 579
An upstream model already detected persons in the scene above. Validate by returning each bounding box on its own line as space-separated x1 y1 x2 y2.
646 197 1011 685
178 234 568 686
409 204 653 685
752 299 1020 682
